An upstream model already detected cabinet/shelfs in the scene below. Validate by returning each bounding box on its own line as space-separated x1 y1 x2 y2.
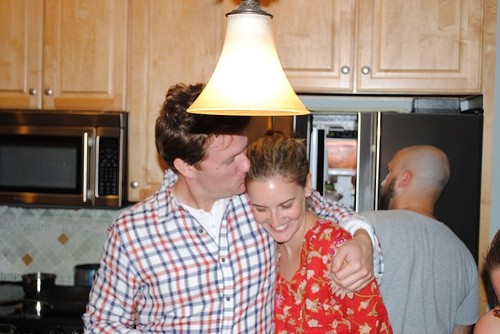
0 0 498 201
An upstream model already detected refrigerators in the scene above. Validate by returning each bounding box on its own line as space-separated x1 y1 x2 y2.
289 93 483 266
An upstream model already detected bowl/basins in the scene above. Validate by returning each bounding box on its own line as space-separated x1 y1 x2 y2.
75 263 100 286
21 272 56 295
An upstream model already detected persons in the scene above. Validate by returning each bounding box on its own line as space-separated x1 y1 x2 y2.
82 83 384 334
244 129 393 334
474 229 500 334
357 144 480 334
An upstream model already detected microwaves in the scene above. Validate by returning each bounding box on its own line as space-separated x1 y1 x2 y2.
0 107 128 211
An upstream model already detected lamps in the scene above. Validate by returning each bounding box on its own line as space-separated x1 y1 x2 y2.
186 0 311 116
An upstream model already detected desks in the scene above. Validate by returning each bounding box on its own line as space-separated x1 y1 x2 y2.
0 280 83 334
